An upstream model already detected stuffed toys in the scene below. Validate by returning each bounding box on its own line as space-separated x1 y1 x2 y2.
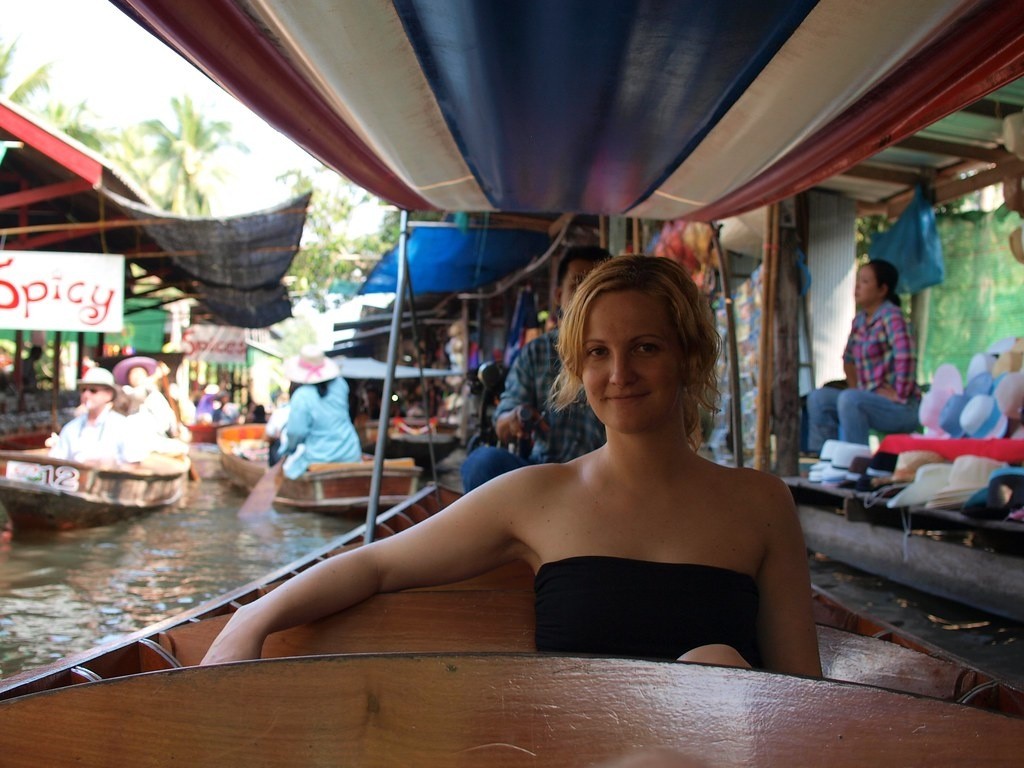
918 336 1024 440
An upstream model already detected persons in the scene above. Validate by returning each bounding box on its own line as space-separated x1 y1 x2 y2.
799 258 922 458
48 356 197 472
195 383 236 424
267 346 362 480
199 255 822 681
10 346 43 392
459 245 615 493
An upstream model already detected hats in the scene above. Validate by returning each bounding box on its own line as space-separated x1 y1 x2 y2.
1001 109 1024 160
281 345 340 384
1010 225 1024 263
204 384 220 394
808 337 1024 520
112 356 157 385
76 367 121 393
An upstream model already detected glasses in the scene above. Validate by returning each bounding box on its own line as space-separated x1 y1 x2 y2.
80 387 109 394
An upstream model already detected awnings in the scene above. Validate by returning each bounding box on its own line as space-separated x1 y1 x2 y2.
95 190 311 328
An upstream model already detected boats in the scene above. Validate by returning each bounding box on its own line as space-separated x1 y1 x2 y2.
0 447 191 533
216 424 423 514
187 422 265 445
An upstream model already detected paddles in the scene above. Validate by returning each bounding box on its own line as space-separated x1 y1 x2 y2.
158 359 200 480
237 455 287 519
